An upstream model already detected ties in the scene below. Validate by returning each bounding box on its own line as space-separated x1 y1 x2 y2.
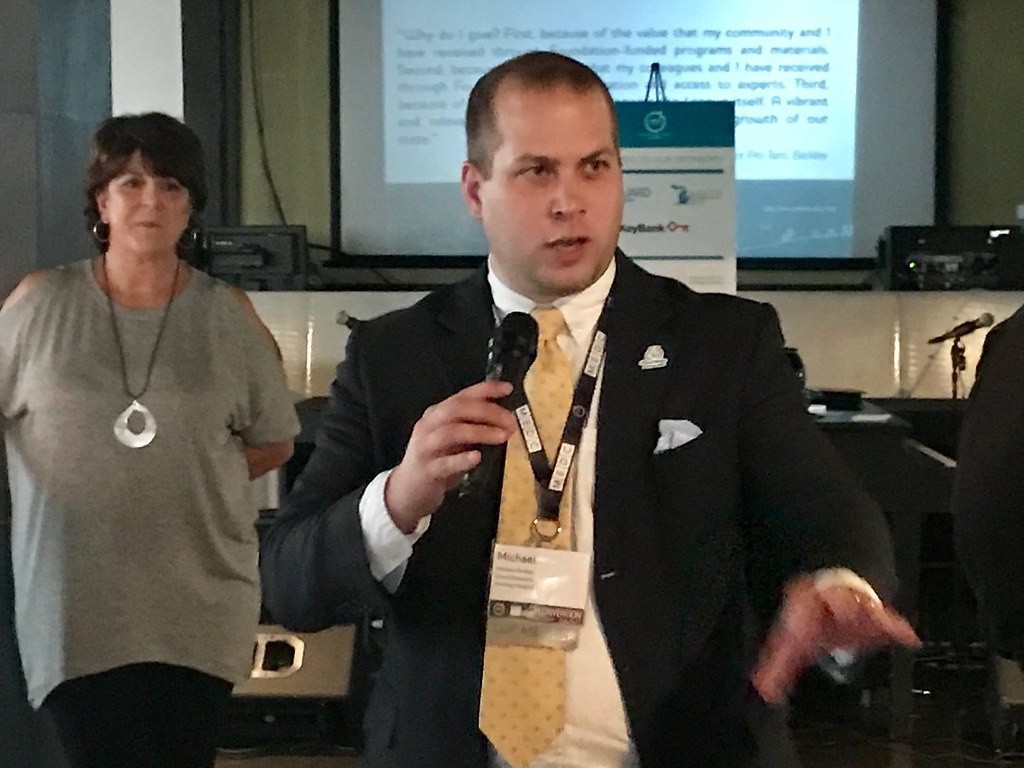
478 307 576 768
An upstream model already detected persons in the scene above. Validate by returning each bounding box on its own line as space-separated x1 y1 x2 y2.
952 304 1024 666
251 49 925 767
0 111 301 768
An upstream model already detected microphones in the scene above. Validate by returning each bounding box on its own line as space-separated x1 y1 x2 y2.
928 314 995 346
461 312 539 500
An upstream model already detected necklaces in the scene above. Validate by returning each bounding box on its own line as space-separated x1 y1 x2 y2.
102 250 181 449
